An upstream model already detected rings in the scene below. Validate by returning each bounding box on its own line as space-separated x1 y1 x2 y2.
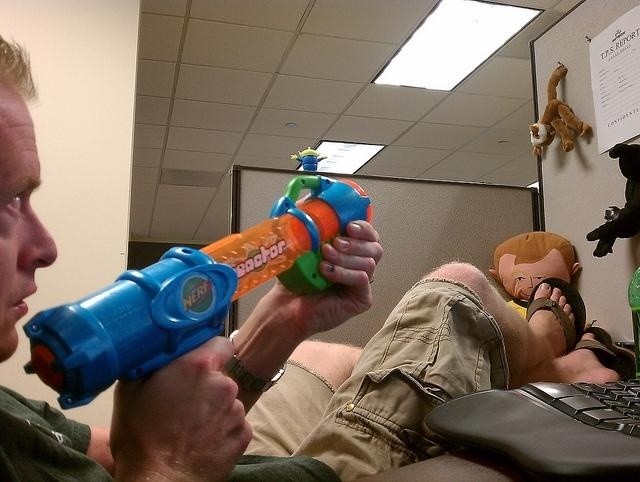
369 274 375 284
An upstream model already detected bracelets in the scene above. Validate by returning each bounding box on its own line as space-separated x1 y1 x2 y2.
224 330 286 392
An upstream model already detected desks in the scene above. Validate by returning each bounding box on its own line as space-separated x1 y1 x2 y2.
351 450 532 481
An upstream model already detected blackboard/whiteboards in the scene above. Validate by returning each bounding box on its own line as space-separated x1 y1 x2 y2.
228 164 540 347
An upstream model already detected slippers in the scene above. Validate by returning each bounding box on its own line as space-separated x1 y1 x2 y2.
526 277 586 356
576 327 636 381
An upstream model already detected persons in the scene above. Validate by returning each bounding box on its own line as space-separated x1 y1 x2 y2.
0 34 637 480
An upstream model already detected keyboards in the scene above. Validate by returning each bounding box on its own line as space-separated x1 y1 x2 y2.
424 379 639 482
